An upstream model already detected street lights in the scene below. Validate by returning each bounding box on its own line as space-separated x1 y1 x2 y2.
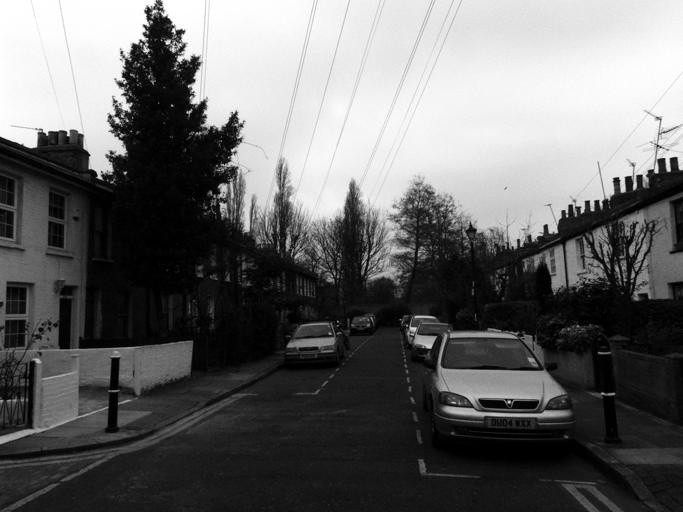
466 221 478 331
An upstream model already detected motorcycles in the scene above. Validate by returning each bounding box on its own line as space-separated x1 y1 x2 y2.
335 318 351 351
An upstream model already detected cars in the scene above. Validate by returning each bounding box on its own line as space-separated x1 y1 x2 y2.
349 313 375 336
398 313 455 361
431 330 577 452
286 321 345 364
